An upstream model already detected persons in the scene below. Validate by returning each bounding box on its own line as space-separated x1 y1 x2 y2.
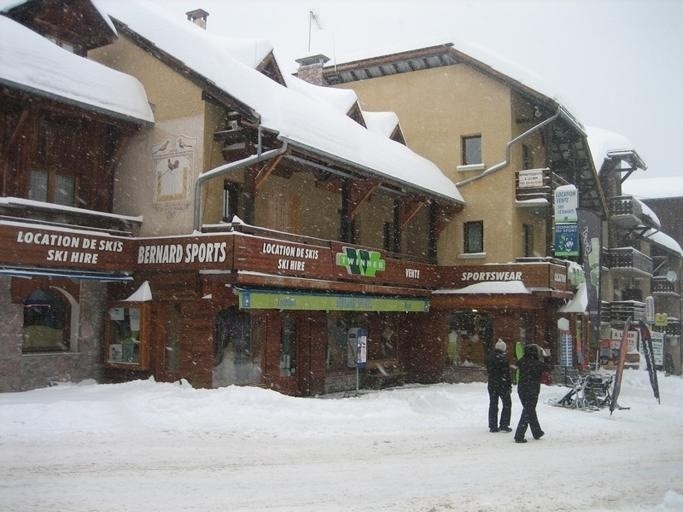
486 338 514 433
512 343 551 443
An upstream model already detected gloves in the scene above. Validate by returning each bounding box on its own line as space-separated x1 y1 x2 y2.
540 348 546 357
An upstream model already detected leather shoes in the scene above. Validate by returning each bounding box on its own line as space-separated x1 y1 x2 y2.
534 431 544 439
490 426 512 432
515 439 527 443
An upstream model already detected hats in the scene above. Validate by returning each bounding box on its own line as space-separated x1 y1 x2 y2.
495 339 506 351
